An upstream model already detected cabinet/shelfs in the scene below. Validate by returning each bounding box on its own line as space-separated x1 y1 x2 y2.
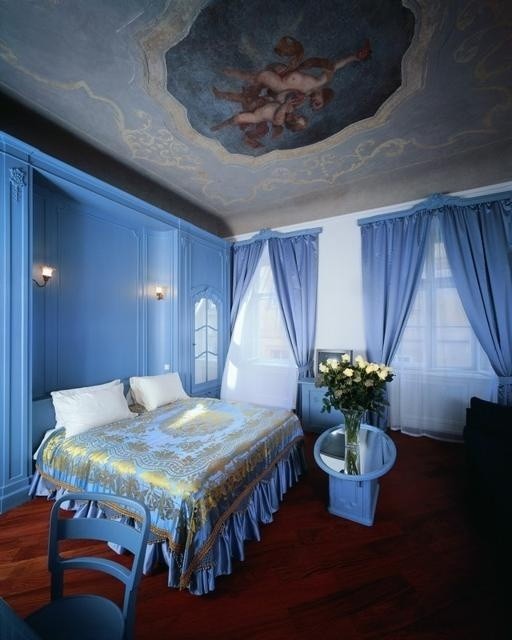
296 377 370 435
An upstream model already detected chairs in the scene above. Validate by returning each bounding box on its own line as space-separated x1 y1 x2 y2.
22 492 150 640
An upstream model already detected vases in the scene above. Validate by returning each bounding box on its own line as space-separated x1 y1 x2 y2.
340 407 368 449
343 445 361 474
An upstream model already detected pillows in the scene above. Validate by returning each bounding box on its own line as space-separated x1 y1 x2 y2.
50 371 190 441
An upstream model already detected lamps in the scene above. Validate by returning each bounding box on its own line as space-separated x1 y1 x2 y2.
33 263 56 288
155 285 164 300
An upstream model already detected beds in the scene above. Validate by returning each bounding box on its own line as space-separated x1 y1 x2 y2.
42 395 300 594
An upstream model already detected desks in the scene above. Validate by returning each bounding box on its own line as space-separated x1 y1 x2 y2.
313 423 397 527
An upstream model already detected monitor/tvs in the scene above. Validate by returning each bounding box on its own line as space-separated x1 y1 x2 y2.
315 348 352 379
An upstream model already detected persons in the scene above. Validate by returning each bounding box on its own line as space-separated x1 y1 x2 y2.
207 36 371 148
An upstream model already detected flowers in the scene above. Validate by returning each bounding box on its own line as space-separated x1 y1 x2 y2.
315 352 397 444
